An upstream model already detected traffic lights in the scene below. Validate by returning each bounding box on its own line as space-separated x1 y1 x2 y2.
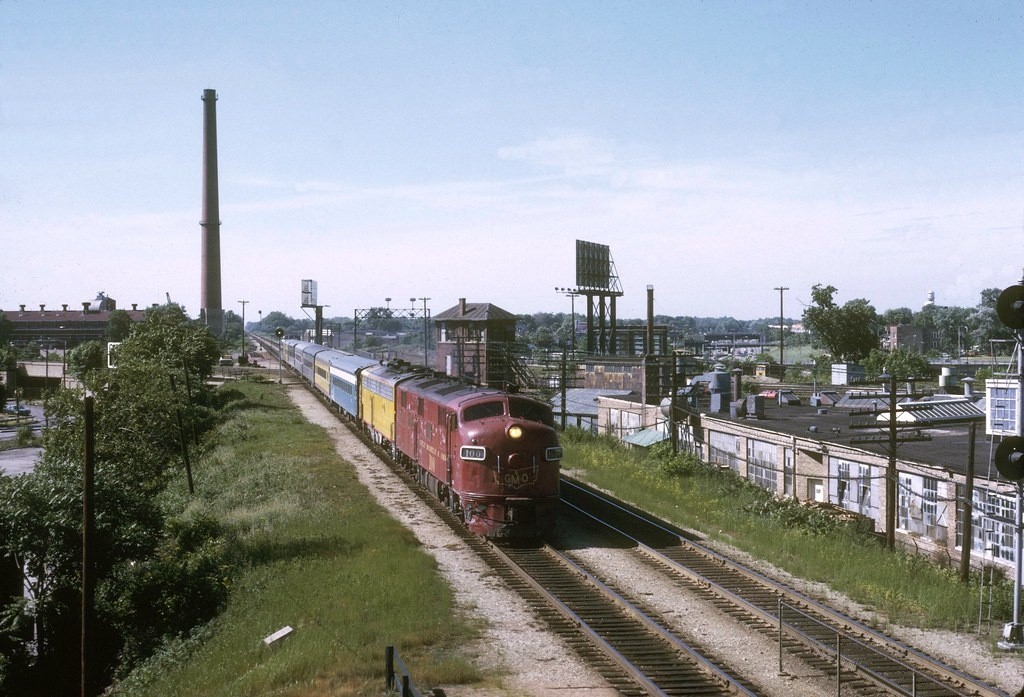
274 328 284 338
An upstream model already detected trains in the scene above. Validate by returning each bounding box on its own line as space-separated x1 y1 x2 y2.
248 328 563 555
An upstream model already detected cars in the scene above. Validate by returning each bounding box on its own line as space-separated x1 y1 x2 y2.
3 404 29 416
